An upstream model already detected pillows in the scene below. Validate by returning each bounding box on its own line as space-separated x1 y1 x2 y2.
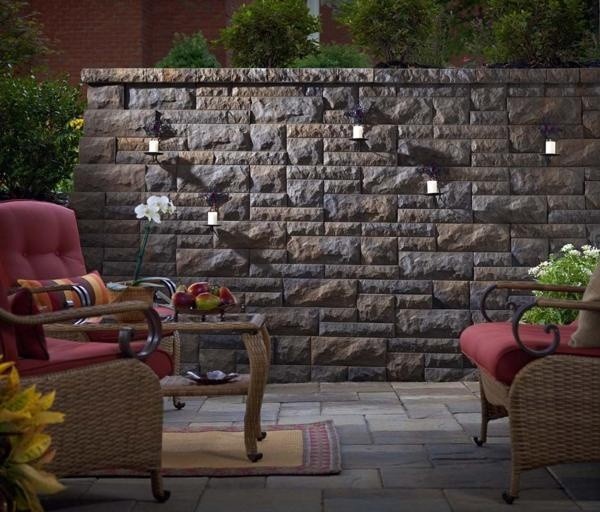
16 269 118 322
567 264 600 348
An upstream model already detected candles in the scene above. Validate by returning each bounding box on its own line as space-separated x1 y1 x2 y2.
148 140 159 153
352 125 363 138
207 211 218 226
426 180 438 194
545 141 556 154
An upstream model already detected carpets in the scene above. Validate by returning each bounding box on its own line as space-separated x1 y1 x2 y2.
55 417 342 477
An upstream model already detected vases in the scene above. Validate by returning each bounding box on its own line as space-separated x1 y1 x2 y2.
105 280 155 323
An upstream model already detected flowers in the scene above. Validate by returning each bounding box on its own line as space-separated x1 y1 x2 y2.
130 195 176 285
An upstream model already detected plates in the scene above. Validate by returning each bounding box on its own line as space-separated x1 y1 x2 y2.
185 372 239 386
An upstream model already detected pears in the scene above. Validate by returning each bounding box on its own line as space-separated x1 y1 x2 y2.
172 282 237 311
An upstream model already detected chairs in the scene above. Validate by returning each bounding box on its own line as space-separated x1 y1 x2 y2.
0 289 170 503
0 201 186 409
458 280 600 502
